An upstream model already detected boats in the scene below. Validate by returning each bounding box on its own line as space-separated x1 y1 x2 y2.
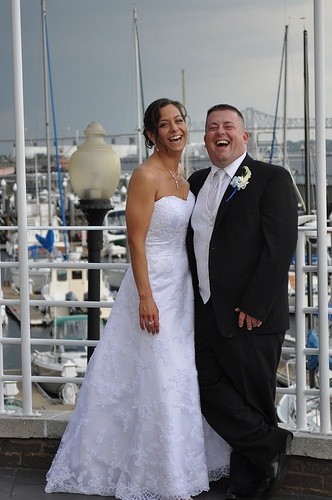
1 170 332 433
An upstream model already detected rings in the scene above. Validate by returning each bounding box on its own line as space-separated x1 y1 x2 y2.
148 321 153 326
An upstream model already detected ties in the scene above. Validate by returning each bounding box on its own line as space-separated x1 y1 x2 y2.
207 170 226 214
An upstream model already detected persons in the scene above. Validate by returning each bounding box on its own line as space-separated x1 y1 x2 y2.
43 97 230 500
186 105 298 499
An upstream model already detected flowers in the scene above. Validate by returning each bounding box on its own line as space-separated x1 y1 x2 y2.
226 166 252 202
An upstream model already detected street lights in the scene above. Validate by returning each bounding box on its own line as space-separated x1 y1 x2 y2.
68 121 121 365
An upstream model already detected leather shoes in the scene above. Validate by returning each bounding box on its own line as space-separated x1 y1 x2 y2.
224 480 252 500
261 433 292 497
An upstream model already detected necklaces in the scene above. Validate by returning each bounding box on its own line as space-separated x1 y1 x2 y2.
153 152 181 189
